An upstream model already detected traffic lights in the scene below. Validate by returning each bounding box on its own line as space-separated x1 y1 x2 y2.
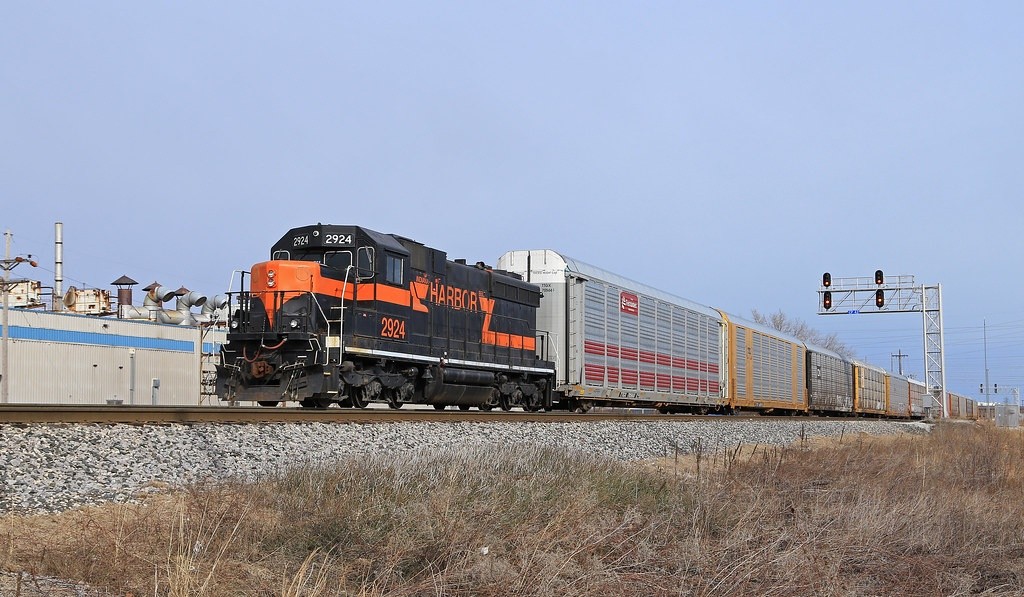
823 272 831 287
876 288 885 307
875 270 884 284
824 291 831 309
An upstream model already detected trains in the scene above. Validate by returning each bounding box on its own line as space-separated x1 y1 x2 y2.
213 220 979 419
